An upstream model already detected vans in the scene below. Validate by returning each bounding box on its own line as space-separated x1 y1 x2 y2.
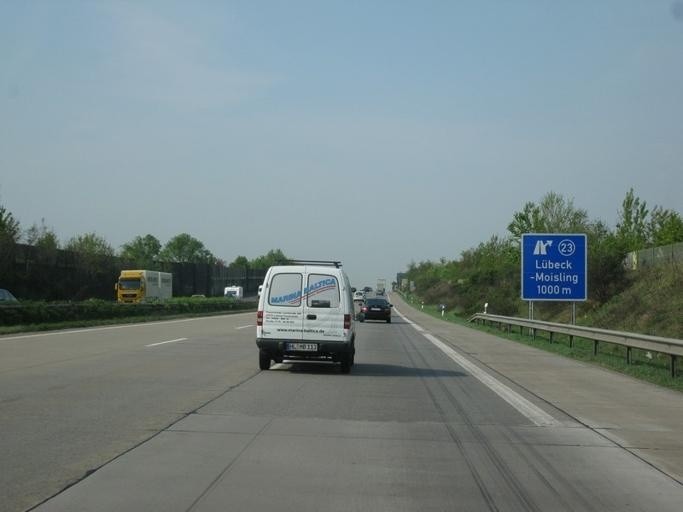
256 285 262 297
254 257 356 376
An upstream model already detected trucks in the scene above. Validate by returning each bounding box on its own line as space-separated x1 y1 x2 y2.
223 286 243 299
113 269 172 305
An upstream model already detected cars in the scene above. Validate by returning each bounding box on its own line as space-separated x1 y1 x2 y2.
350 284 393 323
190 294 205 298
0 289 19 303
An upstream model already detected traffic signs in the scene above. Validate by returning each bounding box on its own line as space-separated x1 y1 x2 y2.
520 234 586 302
